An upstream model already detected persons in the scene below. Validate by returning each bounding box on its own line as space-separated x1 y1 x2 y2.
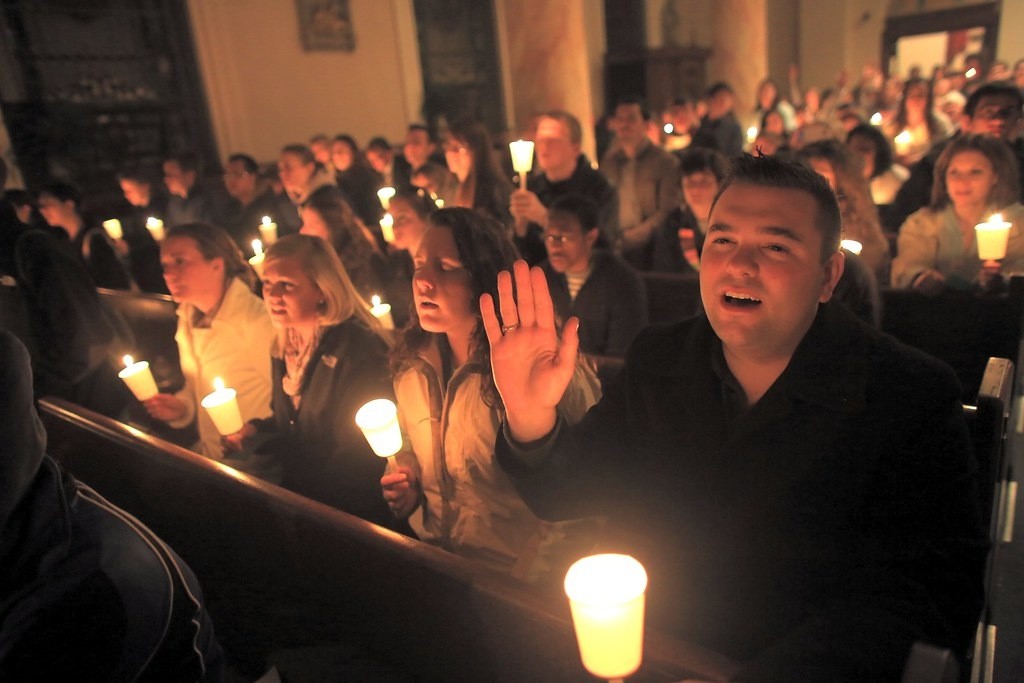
475 154 993 683
1 47 1024 560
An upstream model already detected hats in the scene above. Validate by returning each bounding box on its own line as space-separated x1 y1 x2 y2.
0 326 48 524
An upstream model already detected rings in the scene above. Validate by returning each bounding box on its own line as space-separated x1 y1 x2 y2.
501 324 519 332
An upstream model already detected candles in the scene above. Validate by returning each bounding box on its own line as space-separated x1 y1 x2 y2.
145 218 164 242
509 140 536 190
893 136 910 156
118 360 160 402
258 222 277 246
201 388 244 450
379 218 394 241
355 398 404 474
370 303 395 331
563 553 648 683
248 252 266 282
974 221 1014 262
746 127 757 145
102 218 124 242
377 186 396 211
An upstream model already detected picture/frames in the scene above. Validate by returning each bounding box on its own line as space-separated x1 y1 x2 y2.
294 0 357 53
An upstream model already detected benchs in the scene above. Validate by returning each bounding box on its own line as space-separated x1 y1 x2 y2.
30 392 755 683
96 284 183 387
